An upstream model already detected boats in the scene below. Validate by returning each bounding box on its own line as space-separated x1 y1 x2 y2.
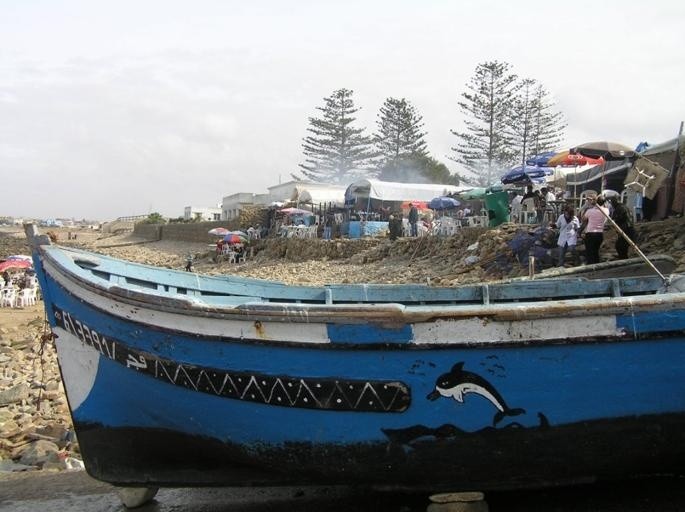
24 221 684 487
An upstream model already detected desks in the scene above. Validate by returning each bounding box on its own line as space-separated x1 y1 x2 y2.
623 155 670 201
547 200 566 221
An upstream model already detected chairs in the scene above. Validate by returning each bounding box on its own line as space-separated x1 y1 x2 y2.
579 189 628 210
216 244 248 264
0 276 38 308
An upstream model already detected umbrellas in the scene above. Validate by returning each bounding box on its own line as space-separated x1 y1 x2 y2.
513 178 546 185
208 227 249 243
526 151 581 185
281 207 314 216
547 150 603 198
0 255 33 272
569 141 641 195
400 199 427 209
428 196 461 217
501 164 553 185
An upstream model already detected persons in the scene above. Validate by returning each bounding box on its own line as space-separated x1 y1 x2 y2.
217 239 243 263
409 203 418 237
3 270 38 293
509 185 639 266
388 214 402 242
184 250 194 272
325 211 334 240
